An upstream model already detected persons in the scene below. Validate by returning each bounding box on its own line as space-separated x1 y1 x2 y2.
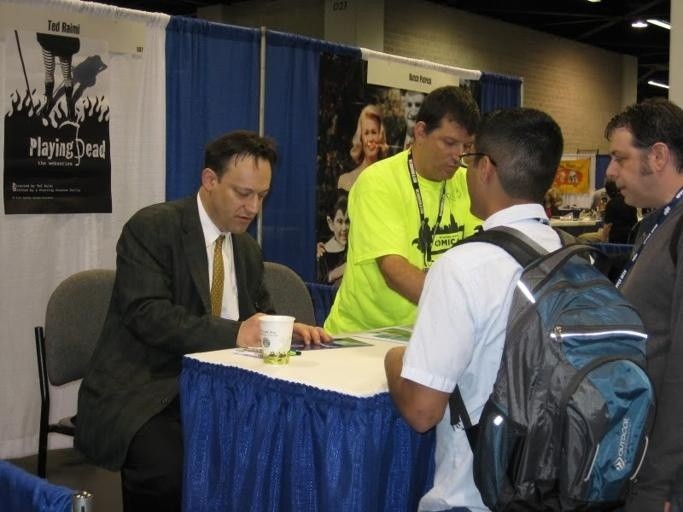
575 198 637 245
542 189 564 218
390 90 426 158
316 105 389 262
320 84 484 337
75 128 333 511
385 107 652 512
319 188 349 283
35 34 79 124
605 105 680 511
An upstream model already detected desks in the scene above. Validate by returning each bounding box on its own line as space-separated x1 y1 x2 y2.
549 215 605 233
178 327 436 512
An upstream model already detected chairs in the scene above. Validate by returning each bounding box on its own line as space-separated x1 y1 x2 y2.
263 262 316 330
27 263 116 480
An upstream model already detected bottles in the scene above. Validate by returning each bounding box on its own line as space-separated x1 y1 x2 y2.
598 191 608 216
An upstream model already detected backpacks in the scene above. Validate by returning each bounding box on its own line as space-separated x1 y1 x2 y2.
449 226 655 511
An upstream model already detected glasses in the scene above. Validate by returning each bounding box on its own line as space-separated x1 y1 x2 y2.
459 153 498 168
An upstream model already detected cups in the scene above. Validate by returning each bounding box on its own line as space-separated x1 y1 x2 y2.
258 315 295 361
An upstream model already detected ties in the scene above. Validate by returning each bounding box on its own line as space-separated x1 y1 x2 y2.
211 235 224 316
